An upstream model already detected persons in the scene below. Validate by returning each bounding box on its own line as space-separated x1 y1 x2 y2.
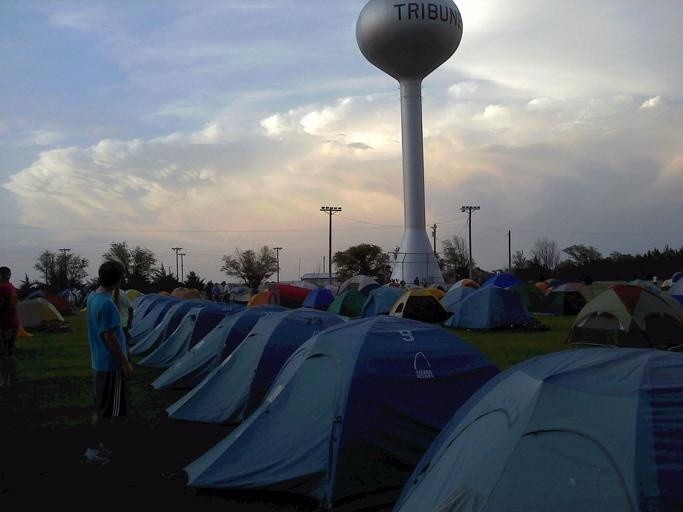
79 259 134 467
106 286 133 362
213 283 222 302
220 281 229 302
0 266 19 394
205 283 214 300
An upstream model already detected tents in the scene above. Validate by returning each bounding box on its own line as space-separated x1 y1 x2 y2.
13 282 97 338
560 282 682 353
267 271 683 332
392 346 682 511
123 287 350 426
180 314 503 512
